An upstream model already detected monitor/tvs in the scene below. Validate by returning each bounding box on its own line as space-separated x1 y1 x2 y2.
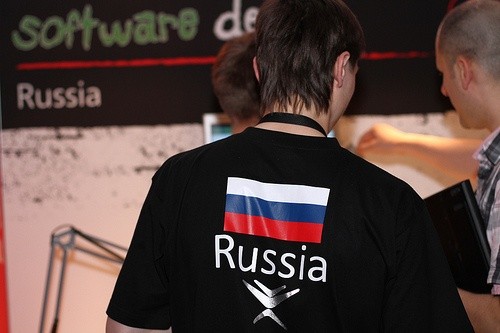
203 113 334 145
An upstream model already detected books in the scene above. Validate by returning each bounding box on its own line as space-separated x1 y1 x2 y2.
423 179 493 295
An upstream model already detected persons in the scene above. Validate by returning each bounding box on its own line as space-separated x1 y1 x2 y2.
211 31 262 134
104 0 477 333
356 0 500 333
